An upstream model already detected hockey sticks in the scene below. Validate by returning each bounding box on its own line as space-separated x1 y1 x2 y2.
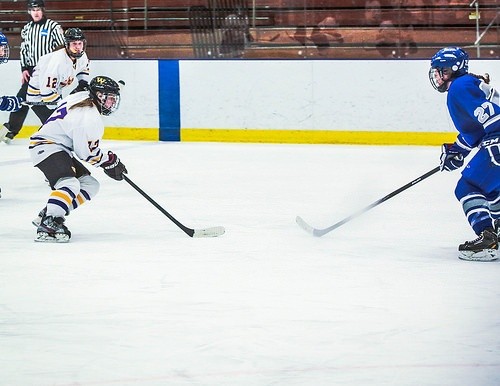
295 166 440 240
18 101 59 106
122 175 226 239
253 33 280 41
329 30 355 42
360 39 412 52
285 30 294 40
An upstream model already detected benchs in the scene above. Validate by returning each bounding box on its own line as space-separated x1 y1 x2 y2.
0 0 500 60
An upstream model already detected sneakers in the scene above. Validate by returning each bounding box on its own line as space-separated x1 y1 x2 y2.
35 216 71 242
3 133 14 144
32 207 68 229
458 230 500 262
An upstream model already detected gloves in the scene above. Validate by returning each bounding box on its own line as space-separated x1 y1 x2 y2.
4 95 24 113
440 144 464 171
100 154 127 180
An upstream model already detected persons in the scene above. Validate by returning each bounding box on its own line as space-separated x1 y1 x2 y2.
428 45 500 261
29 76 129 244
0 0 90 198
218 0 479 58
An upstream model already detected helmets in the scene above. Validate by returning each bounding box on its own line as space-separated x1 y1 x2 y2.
0 32 9 64
88 76 120 116
27 0 45 7
429 46 468 92
64 28 87 57
